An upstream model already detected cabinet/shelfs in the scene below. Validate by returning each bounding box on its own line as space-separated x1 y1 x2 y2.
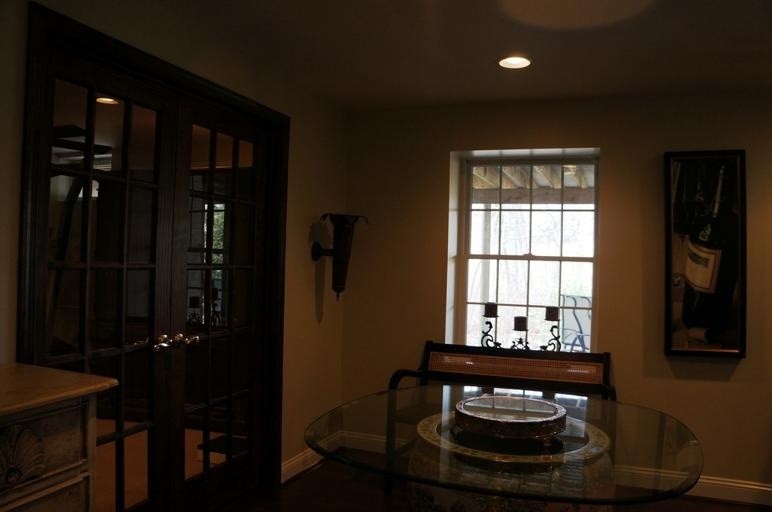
0 358 120 512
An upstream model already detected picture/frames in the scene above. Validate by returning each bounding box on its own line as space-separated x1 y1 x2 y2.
662 148 746 357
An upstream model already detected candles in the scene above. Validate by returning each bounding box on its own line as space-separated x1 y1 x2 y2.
514 316 526 330
546 307 558 320
485 302 497 316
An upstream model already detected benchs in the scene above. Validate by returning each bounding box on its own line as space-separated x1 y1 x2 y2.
387 339 619 402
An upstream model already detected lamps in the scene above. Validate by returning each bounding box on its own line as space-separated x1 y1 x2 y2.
311 211 370 300
497 55 533 69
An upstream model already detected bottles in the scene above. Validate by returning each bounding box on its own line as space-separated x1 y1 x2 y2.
682 163 740 334
